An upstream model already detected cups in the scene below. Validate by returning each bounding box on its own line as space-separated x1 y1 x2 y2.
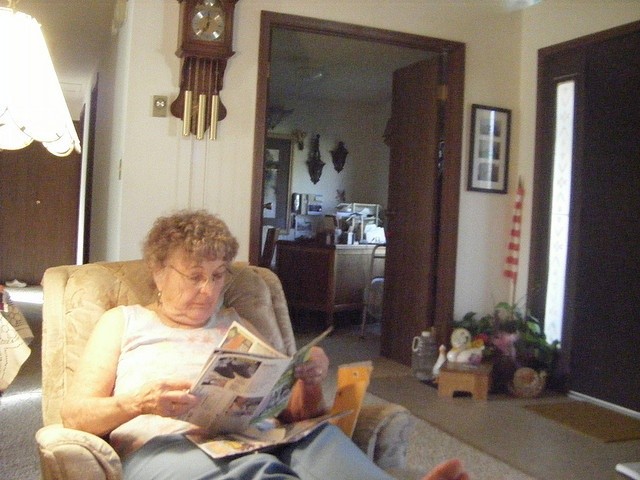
343 231 355 245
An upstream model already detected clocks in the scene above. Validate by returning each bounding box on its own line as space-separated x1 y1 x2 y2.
170 0 236 142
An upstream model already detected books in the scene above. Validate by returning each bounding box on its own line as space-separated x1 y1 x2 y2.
170 319 334 436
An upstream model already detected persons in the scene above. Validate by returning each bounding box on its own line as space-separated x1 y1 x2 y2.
61 210 476 480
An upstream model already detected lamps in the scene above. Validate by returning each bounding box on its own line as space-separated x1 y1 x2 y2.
0 9 85 165
330 141 348 172
307 134 325 185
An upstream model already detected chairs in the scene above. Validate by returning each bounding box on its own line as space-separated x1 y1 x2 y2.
276 239 388 334
260 226 281 265
360 245 386 336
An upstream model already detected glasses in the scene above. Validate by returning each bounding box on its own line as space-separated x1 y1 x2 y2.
169 265 234 288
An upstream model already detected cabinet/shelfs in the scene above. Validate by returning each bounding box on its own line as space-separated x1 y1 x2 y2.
337 203 384 231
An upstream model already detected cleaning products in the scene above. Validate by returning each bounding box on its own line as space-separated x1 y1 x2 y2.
432 344 446 377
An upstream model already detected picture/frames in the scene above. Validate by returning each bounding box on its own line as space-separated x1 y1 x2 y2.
466 104 511 193
262 131 295 234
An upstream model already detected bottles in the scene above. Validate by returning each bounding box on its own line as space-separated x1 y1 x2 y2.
456 344 486 365
432 345 447 383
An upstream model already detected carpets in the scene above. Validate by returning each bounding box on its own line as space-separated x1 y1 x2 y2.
363 373 638 479
522 395 640 444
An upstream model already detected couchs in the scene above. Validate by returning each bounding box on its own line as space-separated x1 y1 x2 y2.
34 259 412 480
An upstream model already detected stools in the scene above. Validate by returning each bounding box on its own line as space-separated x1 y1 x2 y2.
437 360 494 400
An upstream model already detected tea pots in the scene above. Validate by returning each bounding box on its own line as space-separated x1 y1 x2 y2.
323 215 338 234
